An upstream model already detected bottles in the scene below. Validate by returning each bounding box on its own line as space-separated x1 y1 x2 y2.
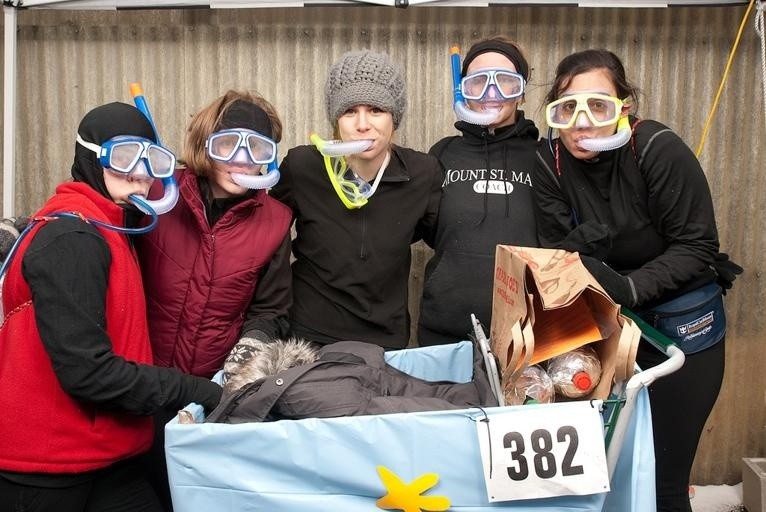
507 347 600 411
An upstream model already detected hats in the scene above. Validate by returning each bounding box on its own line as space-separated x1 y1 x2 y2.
325 46 410 130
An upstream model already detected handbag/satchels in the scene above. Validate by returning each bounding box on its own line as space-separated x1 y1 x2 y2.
645 282 726 356
487 244 640 407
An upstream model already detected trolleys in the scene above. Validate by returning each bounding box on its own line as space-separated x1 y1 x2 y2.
164 308 686 512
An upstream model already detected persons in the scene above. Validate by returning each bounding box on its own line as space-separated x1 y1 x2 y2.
424 31 547 364
530 47 728 512
265 51 449 353
0 98 226 511
106 89 297 493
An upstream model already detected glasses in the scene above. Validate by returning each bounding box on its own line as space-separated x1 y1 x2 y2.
545 92 630 131
204 126 279 168
95 136 179 183
458 68 528 105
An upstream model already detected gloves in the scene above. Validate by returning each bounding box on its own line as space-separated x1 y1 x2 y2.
221 329 273 391
177 368 225 407
581 253 635 312
695 251 744 297
